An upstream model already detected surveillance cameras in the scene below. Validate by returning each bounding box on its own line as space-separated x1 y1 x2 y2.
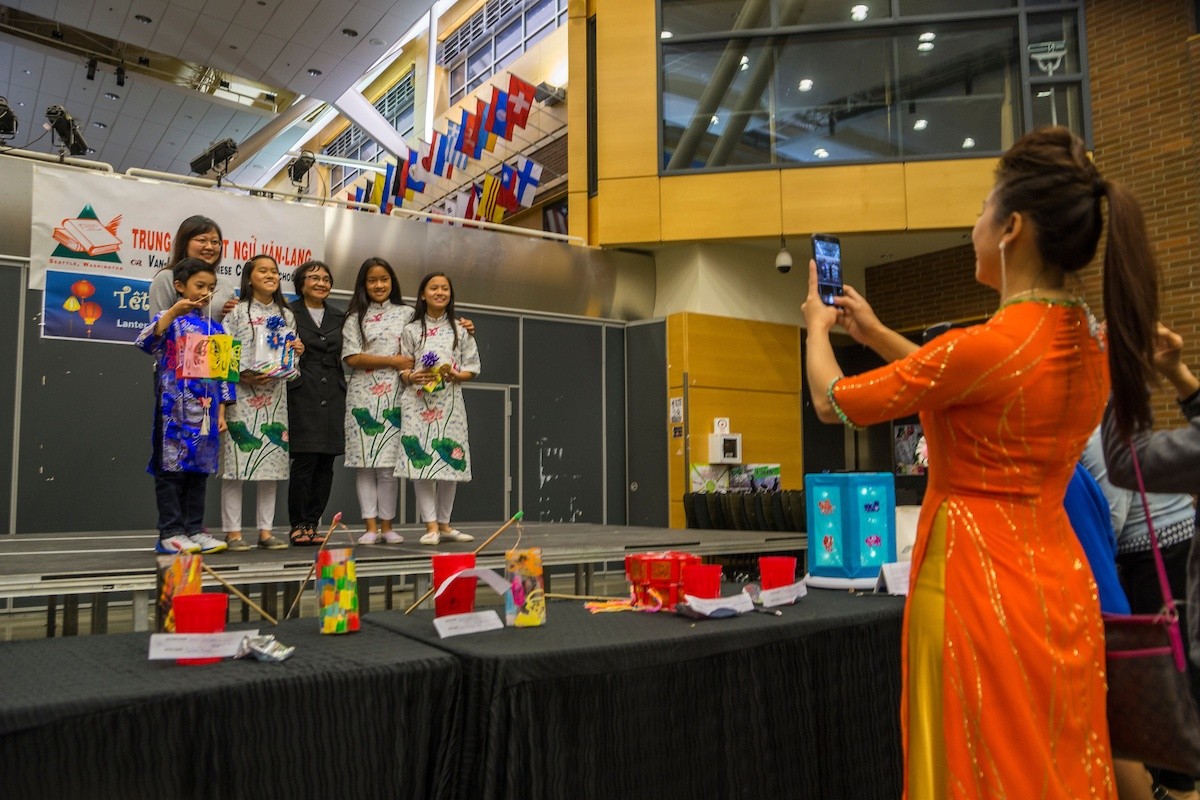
776 252 793 273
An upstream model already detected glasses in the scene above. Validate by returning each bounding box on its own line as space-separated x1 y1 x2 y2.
191 237 223 249
304 274 332 285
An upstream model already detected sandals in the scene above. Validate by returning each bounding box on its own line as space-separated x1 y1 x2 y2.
290 526 309 544
304 525 327 543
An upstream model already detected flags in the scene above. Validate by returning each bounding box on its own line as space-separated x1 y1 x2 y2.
331 70 544 229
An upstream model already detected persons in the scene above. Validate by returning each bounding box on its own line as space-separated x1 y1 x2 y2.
816 258 834 282
800 124 1160 800
134 257 237 554
394 271 481 545
149 216 304 356
916 435 928 477
220 254 305 551
341 256 475 545
1063 321 1200 800
289 260 348 545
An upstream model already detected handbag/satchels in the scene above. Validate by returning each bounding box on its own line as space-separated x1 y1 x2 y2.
1096 610 1200 771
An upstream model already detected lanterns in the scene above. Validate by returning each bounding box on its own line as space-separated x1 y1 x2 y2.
63 279 103 338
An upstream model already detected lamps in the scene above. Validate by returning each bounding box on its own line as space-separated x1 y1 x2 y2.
86 56 99 80
287 148 319 189
0 94 20 140
42 102 90 157
116 62 125 87
190 135 239 176
535 81 565 107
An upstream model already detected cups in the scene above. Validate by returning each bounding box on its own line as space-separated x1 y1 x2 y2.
681 563 722 603
173 592 229 666
759 556 796 589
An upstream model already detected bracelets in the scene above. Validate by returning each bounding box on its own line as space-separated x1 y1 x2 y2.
408 372 414 387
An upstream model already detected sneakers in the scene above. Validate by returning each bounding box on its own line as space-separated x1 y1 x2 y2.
156 535 201 553
190 533 229 552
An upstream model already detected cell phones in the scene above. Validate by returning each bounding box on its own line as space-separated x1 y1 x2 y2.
811 232 844 310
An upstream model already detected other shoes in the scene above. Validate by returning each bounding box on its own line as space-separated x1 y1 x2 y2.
440 529 475 542
379 530 404 543
258 535 289 548
358 531 381 545
223 536 251 551
419 531 442 545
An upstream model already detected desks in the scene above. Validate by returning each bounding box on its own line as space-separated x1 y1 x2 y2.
362 578 907 800
0 613 466 800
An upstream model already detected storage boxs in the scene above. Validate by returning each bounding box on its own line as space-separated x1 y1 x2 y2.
624 550 722 616
805 471 899 576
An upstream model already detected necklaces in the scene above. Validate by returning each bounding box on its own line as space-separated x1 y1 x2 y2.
1004 287 1061 304
